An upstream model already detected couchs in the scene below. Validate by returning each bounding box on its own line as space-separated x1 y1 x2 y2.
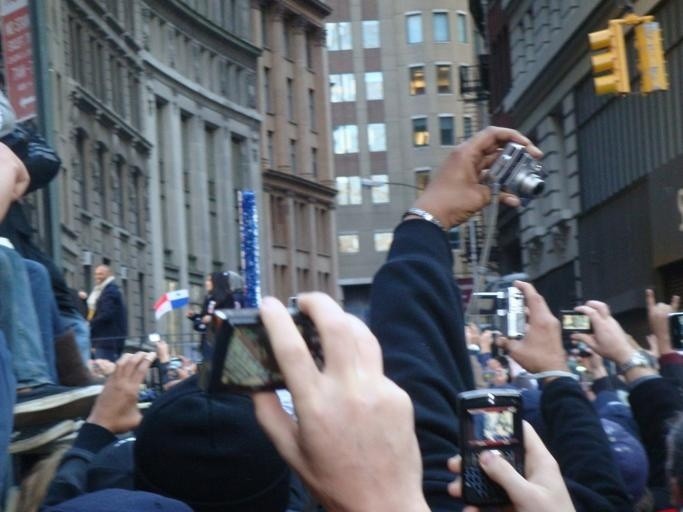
588 26 631 95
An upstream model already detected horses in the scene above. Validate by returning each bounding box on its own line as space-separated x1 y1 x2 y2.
457 389 525 505
134 362 163 415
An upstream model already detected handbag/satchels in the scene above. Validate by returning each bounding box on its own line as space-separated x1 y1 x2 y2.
400 207 445 230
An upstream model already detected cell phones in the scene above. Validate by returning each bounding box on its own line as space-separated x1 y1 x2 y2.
560 309 594 334
469 287 526 338
669 312 683 351
486 143 547 199
200 307 326 392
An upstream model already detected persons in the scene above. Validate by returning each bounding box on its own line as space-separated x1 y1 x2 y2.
84 263 127 362
366 120 636 511
1 273 681 511
0 87 64 510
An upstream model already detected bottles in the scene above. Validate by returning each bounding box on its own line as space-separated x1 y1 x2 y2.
601 416 651 505
130 370 293 511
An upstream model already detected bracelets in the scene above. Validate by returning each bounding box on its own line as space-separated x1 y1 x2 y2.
400 207 445 230
